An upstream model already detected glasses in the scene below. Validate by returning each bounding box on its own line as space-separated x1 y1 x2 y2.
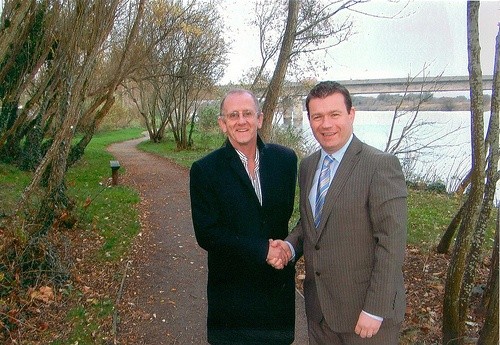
219 111 260 119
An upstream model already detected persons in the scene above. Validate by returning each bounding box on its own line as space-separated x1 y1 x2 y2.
189 89 298 345
267 81 407 345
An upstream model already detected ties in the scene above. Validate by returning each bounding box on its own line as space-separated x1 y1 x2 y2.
313 153 333 230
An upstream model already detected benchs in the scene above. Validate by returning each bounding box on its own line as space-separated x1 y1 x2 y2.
110 160 120 186
